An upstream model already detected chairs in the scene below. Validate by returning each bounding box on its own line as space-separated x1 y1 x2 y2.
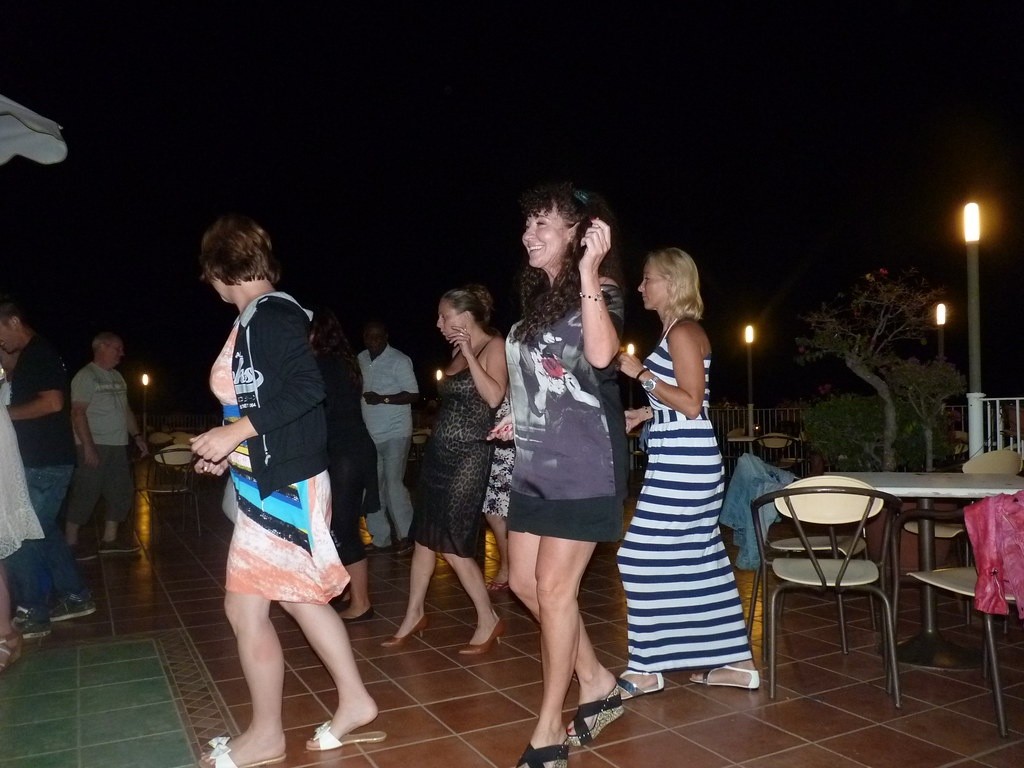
712 424 812 479
749 475 904 711
905 491 1024 738
905 450 1023 631
403 427 433 484
742 450 878 656
135 432 204 548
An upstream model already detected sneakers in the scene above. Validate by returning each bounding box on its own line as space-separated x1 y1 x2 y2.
99 536 141 553
12 609 51 640
69 541 97 560
50 595 96 622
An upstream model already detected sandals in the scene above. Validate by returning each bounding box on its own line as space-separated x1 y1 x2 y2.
616 669 665 700
487 580 511 592
689 665 760 690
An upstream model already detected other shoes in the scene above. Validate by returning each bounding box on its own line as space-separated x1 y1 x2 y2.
341 606 374 624
363 537 413 556
334 597 351 611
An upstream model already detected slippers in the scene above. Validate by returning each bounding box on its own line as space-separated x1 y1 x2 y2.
198 737 286 768
306 720 387 751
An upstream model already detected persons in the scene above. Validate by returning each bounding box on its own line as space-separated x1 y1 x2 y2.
487 185 631 768
616 249 760 700
0 301 97 675
381 284 509 658
64 330 150 563
186 215 386 768
482 380 511 592
359 323 423 555
307 305 376 624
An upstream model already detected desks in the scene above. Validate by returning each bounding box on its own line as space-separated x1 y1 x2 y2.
820 467 1024 669
726 435 800 474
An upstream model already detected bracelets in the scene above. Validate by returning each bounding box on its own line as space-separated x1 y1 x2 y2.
133 433 142 438
637 367 650 379
641 405 651 419
579 289 602 303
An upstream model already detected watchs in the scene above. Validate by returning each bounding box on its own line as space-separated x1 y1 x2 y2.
642 377 659 392
384 395 390 404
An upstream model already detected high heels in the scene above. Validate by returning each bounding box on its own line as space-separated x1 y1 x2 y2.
459 617 506 656
566 683 625 747
0 629 23 673
383 614 428 645
514 736 570 768
